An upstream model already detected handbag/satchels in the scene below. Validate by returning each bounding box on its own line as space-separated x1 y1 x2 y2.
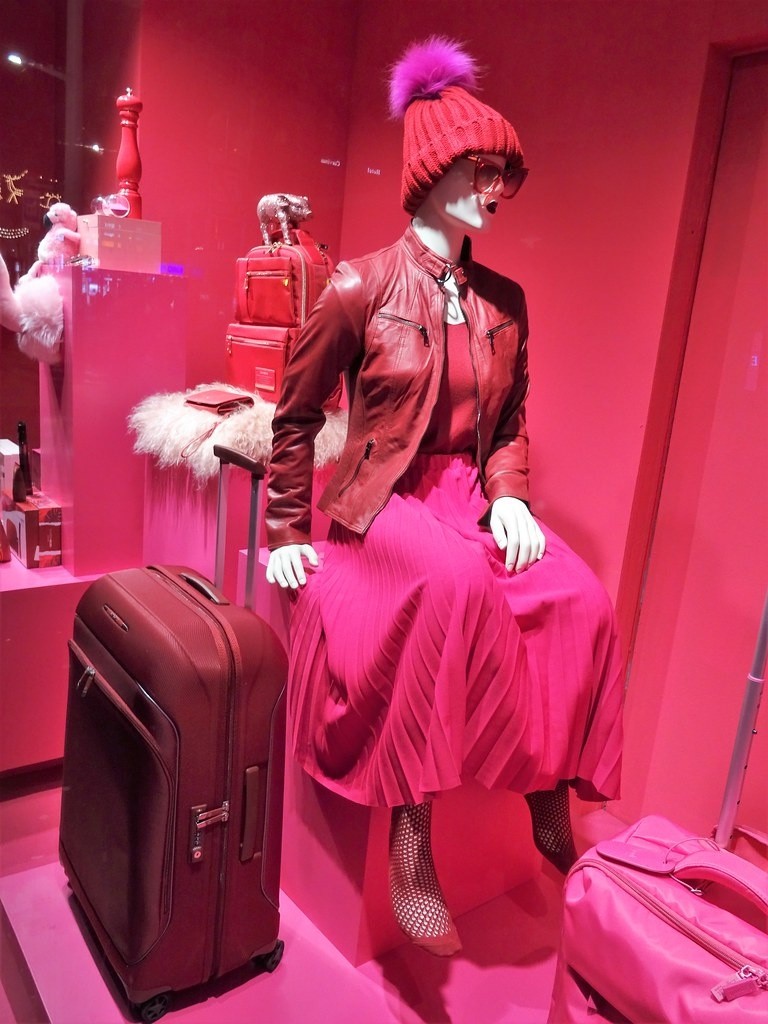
224 211 343 326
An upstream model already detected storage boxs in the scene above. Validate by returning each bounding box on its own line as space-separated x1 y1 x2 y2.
0 439 63 569
77 214 162 273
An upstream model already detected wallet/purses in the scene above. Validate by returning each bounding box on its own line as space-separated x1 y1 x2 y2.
179 390 255 458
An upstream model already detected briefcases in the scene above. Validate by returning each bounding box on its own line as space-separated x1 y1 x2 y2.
222 320 342 416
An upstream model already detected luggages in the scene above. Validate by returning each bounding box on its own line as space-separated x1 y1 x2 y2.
546 608 768 1024
57 446 294 1024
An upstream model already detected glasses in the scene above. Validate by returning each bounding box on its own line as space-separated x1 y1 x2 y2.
458 151 530 200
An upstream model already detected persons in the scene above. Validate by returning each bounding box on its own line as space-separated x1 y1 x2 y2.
263 35 625 962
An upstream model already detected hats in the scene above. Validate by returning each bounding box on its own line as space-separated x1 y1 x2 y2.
387 39 526 217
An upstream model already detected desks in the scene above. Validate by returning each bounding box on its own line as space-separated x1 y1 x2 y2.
238 545 545 962
